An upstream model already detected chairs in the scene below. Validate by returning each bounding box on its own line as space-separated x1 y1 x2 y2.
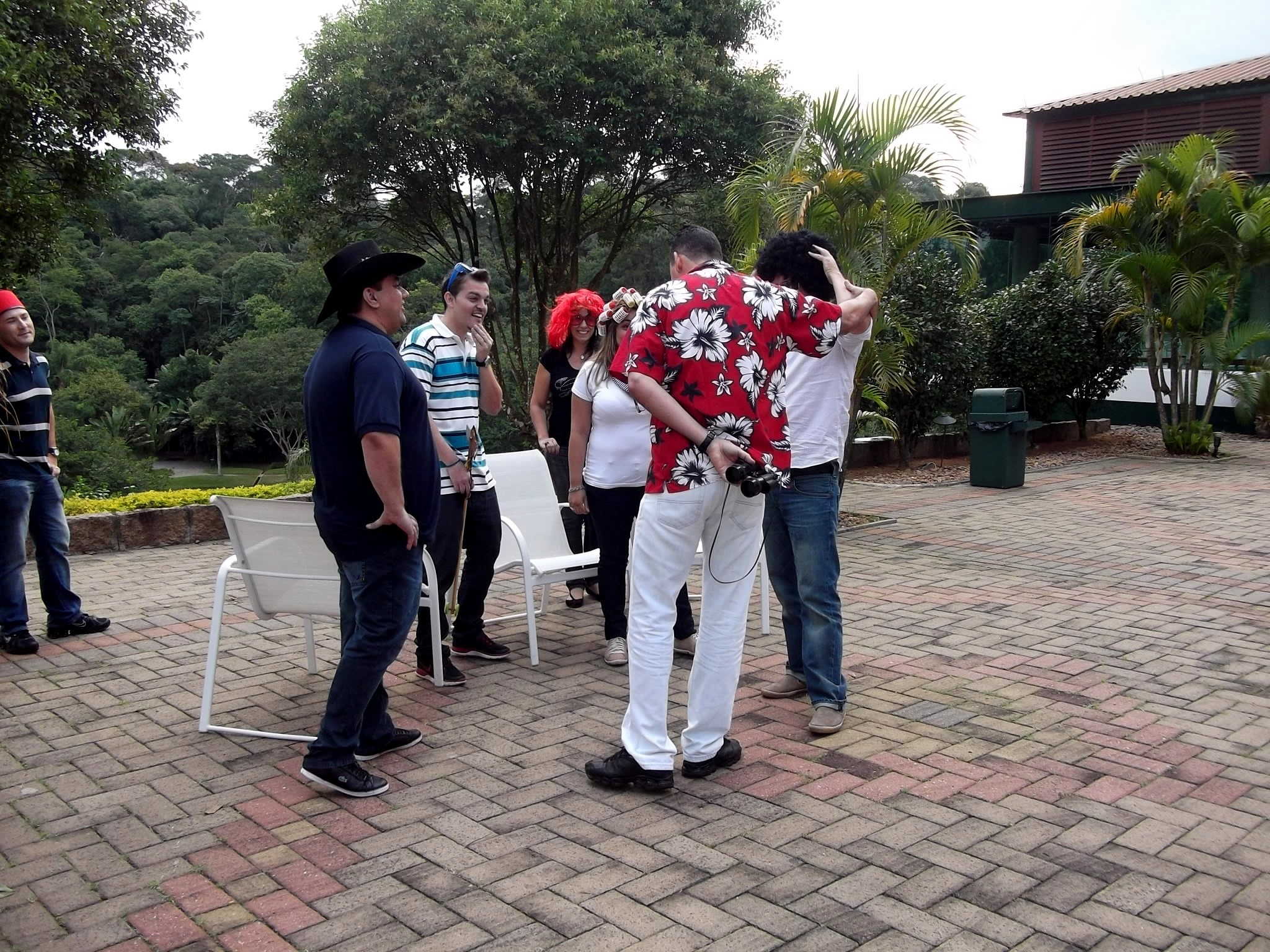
468 450 588 666
687 537 770 636
199 493 442 744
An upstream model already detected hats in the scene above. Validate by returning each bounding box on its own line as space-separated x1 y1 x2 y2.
0 289 26 314
313 239 427 326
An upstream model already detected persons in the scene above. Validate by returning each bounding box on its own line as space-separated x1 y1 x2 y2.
1 288 111 653
395 259 512 686
304 240 440 800
530 289 602 608
584 222 877 791
746 227 875 735
568 286 697 665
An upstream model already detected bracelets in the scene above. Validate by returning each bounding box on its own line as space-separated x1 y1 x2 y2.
569 486 584 494
699 431 716 453
446 459 461 468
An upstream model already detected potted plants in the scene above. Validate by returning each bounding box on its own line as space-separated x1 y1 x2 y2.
1219 354 1270 438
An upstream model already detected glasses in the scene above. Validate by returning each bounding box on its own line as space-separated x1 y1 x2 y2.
774 278 802 290
447 261 480 292
570 315 596 327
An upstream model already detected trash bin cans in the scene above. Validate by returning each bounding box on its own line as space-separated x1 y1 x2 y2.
968 387 1029 489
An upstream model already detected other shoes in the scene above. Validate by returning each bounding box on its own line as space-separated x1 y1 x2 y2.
585 586 601 602
565 587 584 607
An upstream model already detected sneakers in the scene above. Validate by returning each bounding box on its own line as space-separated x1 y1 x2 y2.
673 633 698 655
300 758 389 797
808 705 846 733
760 674 808 698
0 629 40 654
585 748 674 790
681 737 741 778
415 656 467 685
450 635 512 659
354 728 423 761
47 613 110 639
604 637 628 664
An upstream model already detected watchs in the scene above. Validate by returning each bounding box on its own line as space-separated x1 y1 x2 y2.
475 355 492 367
48 445 60 457
